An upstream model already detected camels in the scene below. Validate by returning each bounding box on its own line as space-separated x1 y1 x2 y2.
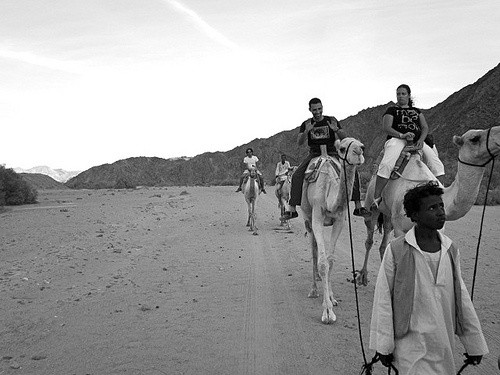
239 168 263 235
275 166 298 233
301 137 365 324
357 127 499 286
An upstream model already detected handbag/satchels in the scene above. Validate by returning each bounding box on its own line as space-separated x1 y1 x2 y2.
424 134 434 148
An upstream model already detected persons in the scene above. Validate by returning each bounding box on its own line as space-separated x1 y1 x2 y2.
367 179 489 375
370 84 445 210
278 98 373 223
235 148 267 194
275 155 292 190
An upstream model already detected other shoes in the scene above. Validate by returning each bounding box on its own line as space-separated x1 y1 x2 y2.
370 197 382 210
281 211 298 220
235 188 242 192
262 188 266 194
353 207 371 217
